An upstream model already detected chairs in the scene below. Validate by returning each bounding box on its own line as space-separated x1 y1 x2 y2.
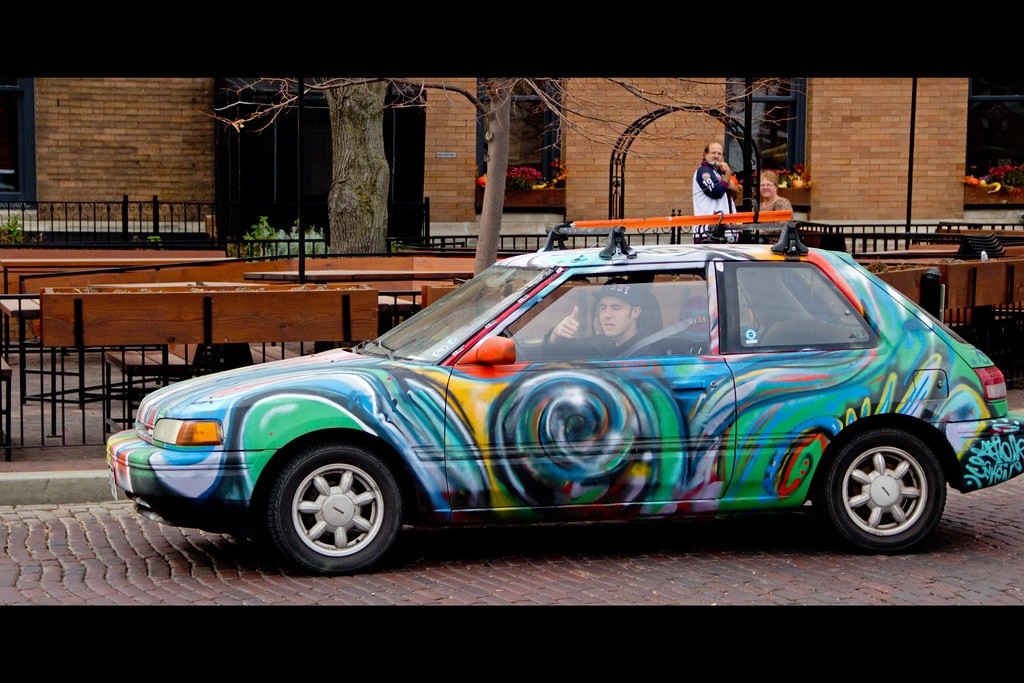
636 286 665 357
103 343 193 434
0 357 13 462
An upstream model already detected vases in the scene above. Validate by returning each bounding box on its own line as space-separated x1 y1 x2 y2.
750 185 811 206
476 184 566 207
964 184 1024 204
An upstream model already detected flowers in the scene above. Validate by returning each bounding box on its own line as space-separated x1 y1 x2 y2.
964 159 1024 193
747 164 810 188
478 158 566 191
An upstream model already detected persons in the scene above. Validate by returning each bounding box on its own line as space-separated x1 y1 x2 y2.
540 278 669 363
692 142 742 242
752 171 794 242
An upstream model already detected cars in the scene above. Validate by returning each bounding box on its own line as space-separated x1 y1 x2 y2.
103 209 1024 575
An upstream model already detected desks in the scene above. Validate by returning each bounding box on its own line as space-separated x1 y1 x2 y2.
0 297 92 437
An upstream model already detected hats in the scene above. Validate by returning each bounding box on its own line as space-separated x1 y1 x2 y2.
592 278 639 305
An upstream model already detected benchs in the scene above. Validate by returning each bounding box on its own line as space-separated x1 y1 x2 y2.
748 319 863 343
